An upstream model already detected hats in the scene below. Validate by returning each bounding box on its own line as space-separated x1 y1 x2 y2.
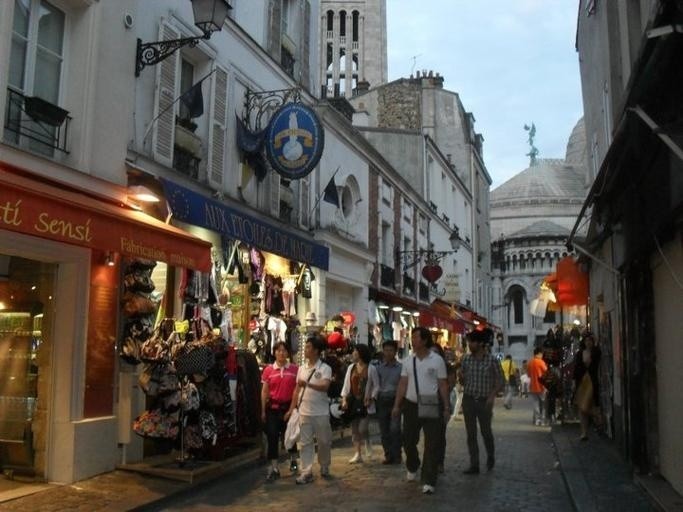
330 403 345 419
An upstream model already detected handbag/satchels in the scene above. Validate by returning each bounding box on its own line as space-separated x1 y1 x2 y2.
165 383 200 411
186 413 218 451
124 295 154 318
141 330 169 364
509 374 516 386
117 333 140 364
173 346 209 374
417 392 438 419
196 318 222 352
124 269 155 292
132 409 178 439
138 366 177 397
351 400 367 417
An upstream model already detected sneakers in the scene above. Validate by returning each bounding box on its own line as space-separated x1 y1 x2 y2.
350 457 361 463
296 474 313 484
423 484 435 493
408 471 417 482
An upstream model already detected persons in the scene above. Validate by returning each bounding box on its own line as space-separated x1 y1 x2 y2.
252 253 263 276
428 344 454 472
457 331 504 473
240 250 250 276
283 337 332 483
260 344 296 482
340 343 379 464
391 326 450 493
441 329 555 426
377 341 402 465
573 335 603 441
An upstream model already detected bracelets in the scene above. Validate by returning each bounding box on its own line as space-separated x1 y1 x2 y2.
305 381 307 387
442 406 450 410
494 387 496 393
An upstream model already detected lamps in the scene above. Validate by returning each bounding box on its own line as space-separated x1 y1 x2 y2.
132 1 235 78
422 231 464 265
125 174 165 206
